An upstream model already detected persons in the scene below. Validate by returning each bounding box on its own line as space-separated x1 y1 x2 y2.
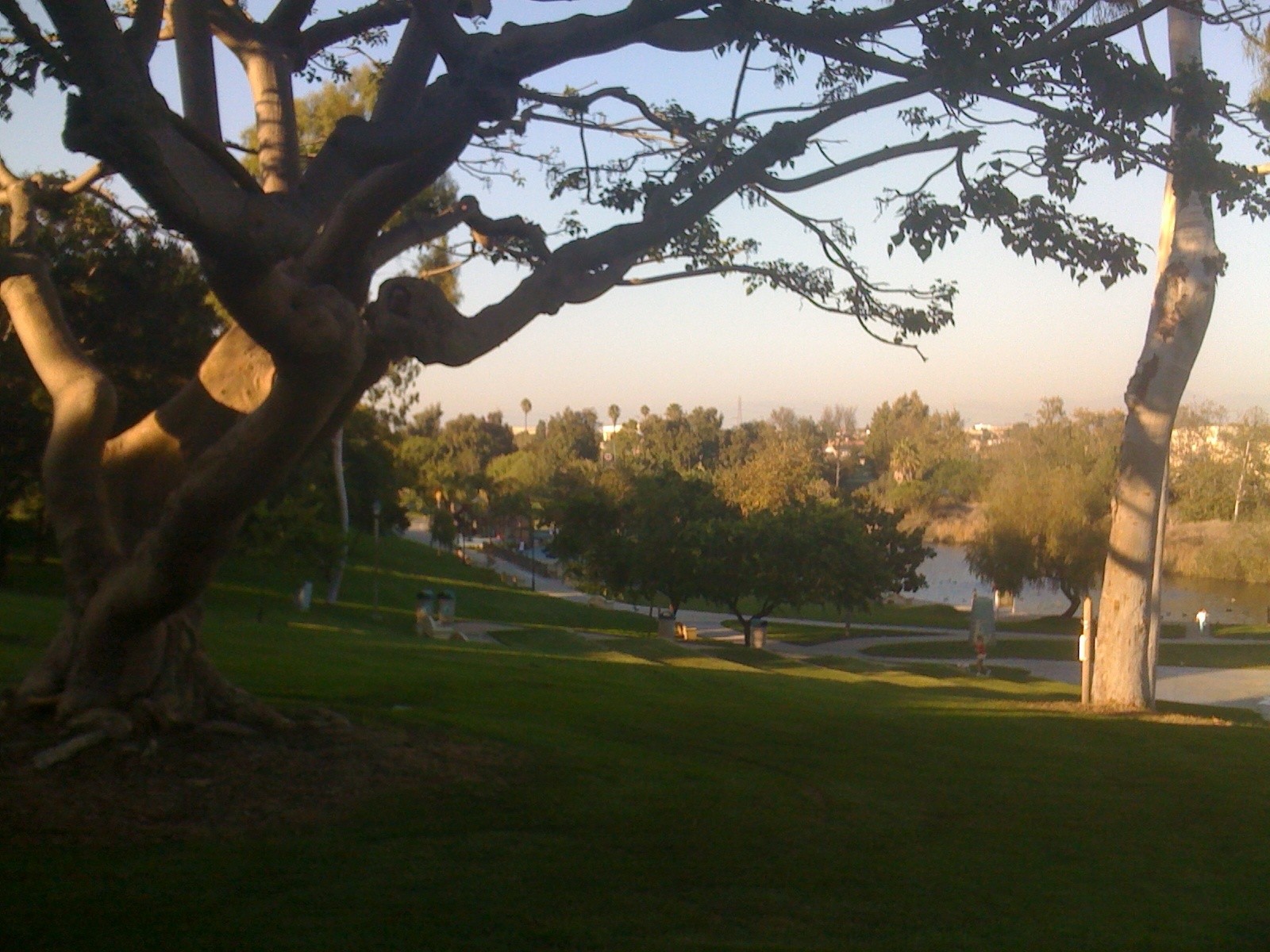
520 539 524 553
1196 608 1207 634
501 571 513 584
454 545 475 565
415 588 468 640
974 633 991 676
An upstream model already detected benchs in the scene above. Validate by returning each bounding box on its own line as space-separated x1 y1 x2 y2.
677 622 697 640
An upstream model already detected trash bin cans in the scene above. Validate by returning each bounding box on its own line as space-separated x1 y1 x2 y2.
418 590 434 617
749 619 769 648
438 589 455 622
658 610 676 640
